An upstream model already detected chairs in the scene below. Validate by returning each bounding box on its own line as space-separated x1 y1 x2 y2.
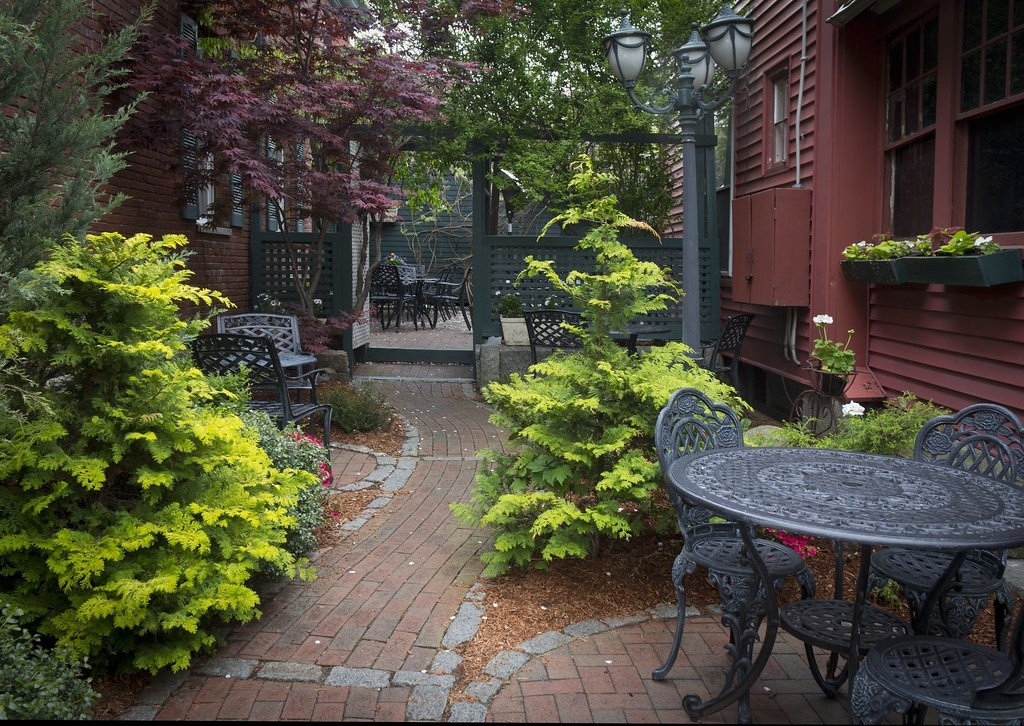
522 309 591 378
371 262 473 332
704 311 756 426
650 386 1024 726
216 310 313 425
193 333 335 487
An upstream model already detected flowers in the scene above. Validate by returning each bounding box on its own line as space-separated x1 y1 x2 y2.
384 252 409 267
896 224 1001 257
840 241 875 260
811 313 858 380
872 239 922 260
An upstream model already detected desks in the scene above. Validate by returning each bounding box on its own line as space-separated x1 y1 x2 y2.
395 277 440 330
607 324 671 356
213 352 319 437
668 445 1024 726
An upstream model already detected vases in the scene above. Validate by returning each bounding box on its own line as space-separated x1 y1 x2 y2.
870 259 903 287
840 260 872 286
896 246 1024 287
821 372 849 395
